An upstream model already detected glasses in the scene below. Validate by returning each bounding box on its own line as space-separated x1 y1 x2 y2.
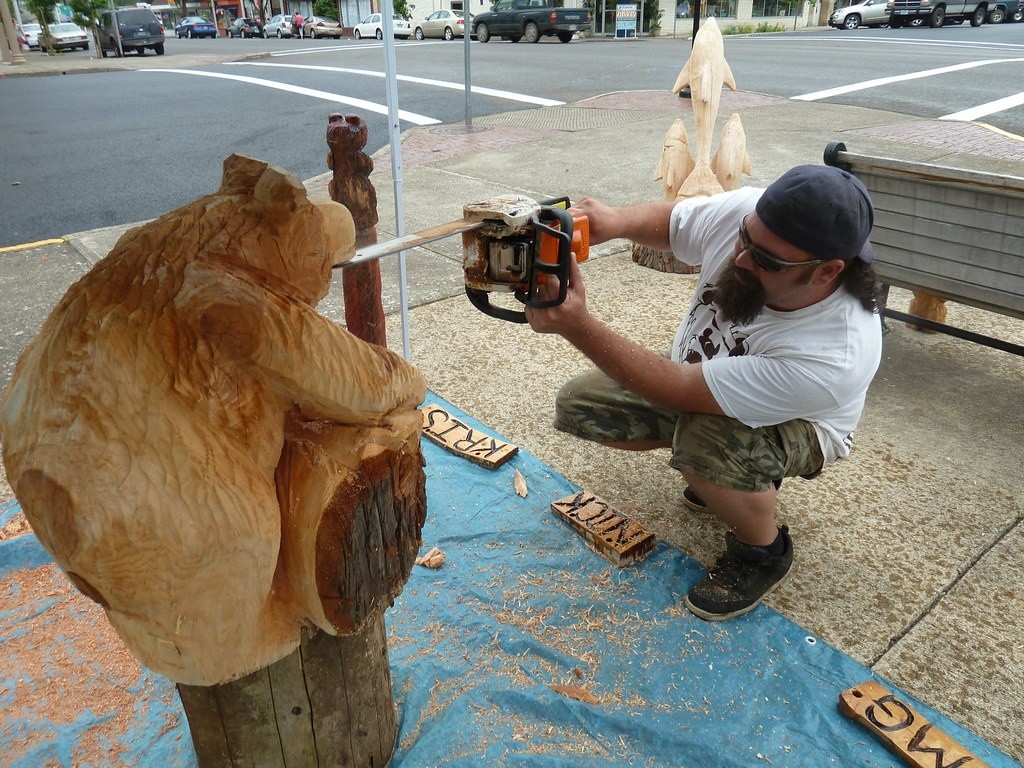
738 213 824 271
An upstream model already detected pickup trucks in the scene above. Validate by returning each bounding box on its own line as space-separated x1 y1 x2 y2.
471 0 594 46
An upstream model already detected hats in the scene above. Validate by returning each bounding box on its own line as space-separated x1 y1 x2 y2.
754 164 876 264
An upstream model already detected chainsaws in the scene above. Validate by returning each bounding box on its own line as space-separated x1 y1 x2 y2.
332 194 589 323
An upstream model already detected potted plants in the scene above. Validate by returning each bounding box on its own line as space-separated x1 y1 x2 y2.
646 0 662 36
582 0 595 37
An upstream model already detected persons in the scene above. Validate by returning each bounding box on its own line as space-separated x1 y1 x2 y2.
524 165 886 621
294 11 304 39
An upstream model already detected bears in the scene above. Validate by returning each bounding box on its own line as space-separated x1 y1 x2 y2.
2 151 427 687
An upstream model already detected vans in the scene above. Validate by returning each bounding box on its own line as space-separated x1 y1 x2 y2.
93 7 166 56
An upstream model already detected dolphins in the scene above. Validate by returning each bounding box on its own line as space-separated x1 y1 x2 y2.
653 16 753 201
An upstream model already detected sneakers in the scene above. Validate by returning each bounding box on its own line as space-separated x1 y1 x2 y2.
685 524 794 621
679 477 782 513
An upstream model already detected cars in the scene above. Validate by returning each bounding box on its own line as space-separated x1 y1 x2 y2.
37 22 91 52
828 0 924 31
945 0 1024 25
174 16 217 39
353 13 412 40
889 0 999 29
261 13 296 39
22 23 43 50
226 17 263 39
289 14 344 40
412 8 477 42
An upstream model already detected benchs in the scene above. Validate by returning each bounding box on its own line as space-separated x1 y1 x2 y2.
824 140 1024 357
